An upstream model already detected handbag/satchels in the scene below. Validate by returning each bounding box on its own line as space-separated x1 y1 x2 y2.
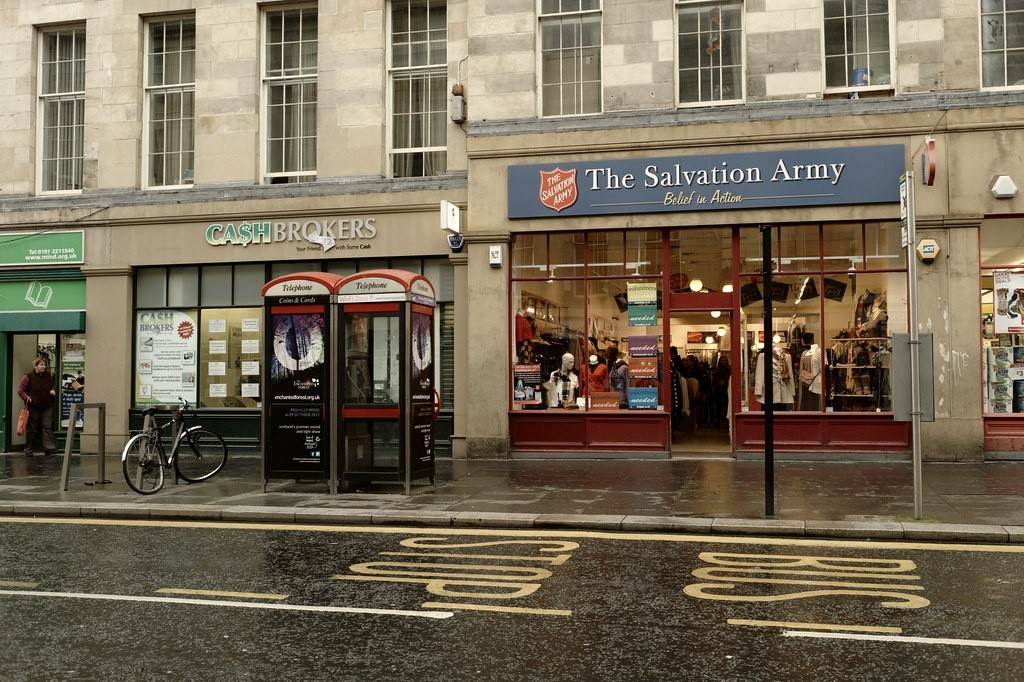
855 351 871 366
16 397 31 436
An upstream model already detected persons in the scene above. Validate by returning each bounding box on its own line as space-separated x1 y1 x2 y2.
76 371 84 393
712 356 732 424
604 346 620 373
798 332 814 361
855 288 876 332
750 344 756 373
754 347 796 411
786 319 801 347
610 359 629 404
867 297 887 321
578 355 611 398
516 308 533 356
549 352 580 409
18 358 59 456
670 346 684 377
798 344 829 410
526 307 536 335
685 354 703 428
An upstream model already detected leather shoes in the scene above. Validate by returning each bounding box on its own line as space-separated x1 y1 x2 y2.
24 448 33 455
44 449 59 455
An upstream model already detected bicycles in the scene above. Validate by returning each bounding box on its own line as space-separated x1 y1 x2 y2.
121 396 230 495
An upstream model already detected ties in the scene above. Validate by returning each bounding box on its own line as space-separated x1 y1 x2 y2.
856 298 863 330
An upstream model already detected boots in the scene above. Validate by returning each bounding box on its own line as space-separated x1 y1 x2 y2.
852 375 872 395
